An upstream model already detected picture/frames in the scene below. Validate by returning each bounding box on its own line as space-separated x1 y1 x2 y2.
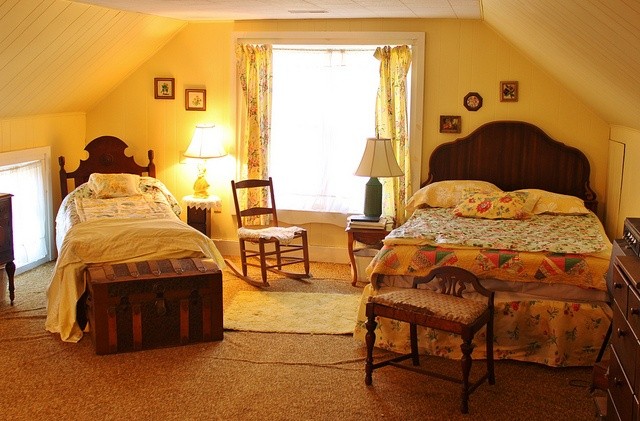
463 92 483 111
154 78 175 99
439 115 461 133
185 89 206 111
500 81 519 103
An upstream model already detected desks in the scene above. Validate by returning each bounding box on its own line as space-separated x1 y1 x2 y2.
0 193 16 305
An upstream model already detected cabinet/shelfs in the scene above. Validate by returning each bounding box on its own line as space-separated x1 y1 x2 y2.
596 217 640 420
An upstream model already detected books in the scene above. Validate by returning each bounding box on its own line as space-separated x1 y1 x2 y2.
349 216 388 230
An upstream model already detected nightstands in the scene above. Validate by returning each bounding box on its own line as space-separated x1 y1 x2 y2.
187 201 211 239
345 218 394 286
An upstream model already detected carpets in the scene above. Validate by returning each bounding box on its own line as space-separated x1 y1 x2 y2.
223 291 362 334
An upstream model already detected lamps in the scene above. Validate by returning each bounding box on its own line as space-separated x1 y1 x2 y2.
184 124 229 198
352 138 406 218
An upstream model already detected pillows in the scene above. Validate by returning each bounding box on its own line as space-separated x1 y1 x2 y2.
405 180 504 210
512 189 590 216
452 189 540 219
88 173 142 198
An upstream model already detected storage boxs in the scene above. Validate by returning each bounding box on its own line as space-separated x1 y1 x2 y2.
85 258 223 355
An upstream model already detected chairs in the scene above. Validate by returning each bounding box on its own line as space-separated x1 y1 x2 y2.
228 178 313 286
365 266 495 413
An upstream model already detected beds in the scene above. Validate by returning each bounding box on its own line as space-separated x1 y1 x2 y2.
355 120 612 367
43 136 224 344
